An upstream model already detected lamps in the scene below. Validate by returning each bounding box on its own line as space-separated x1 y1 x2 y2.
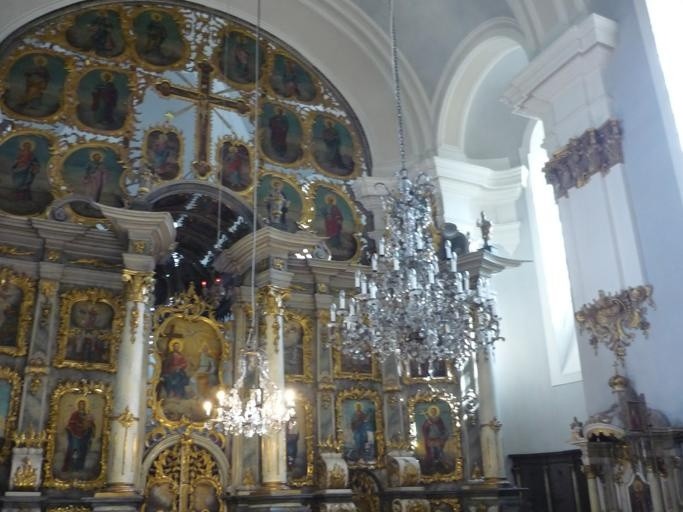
323 0 505 378
